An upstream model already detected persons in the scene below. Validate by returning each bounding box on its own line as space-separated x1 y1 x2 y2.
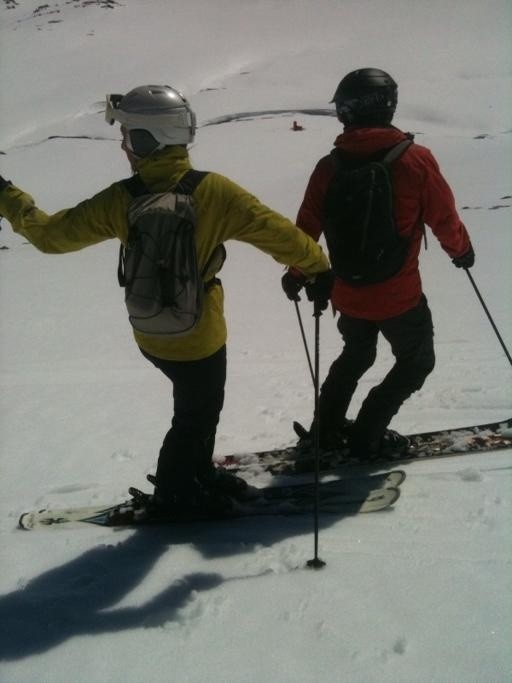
281 70 474 463
1 85 335 524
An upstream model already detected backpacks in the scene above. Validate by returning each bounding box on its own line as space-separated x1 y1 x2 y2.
322 135 420 291
116 170 209 335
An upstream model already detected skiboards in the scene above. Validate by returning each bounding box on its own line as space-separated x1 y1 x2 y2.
19 469 404 530
214 418 511 472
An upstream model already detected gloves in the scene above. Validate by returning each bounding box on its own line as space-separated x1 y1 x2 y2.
303 273 337 311
451 249 476 270
281 272 306 303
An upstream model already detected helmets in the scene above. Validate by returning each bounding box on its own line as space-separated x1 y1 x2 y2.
115 84 195 154
329 67 399 128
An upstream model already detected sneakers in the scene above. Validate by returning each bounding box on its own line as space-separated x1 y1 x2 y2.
308 418 411 460
154 468 248 524
